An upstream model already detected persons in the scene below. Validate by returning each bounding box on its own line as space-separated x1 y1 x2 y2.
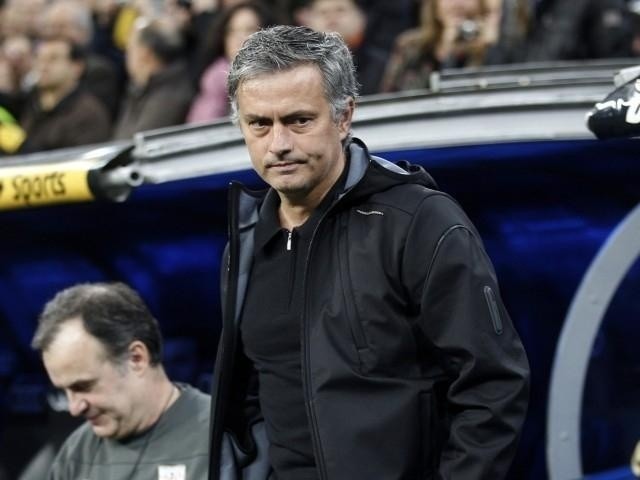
18 282 213 480
0 0 639 155
207 23 532 480
